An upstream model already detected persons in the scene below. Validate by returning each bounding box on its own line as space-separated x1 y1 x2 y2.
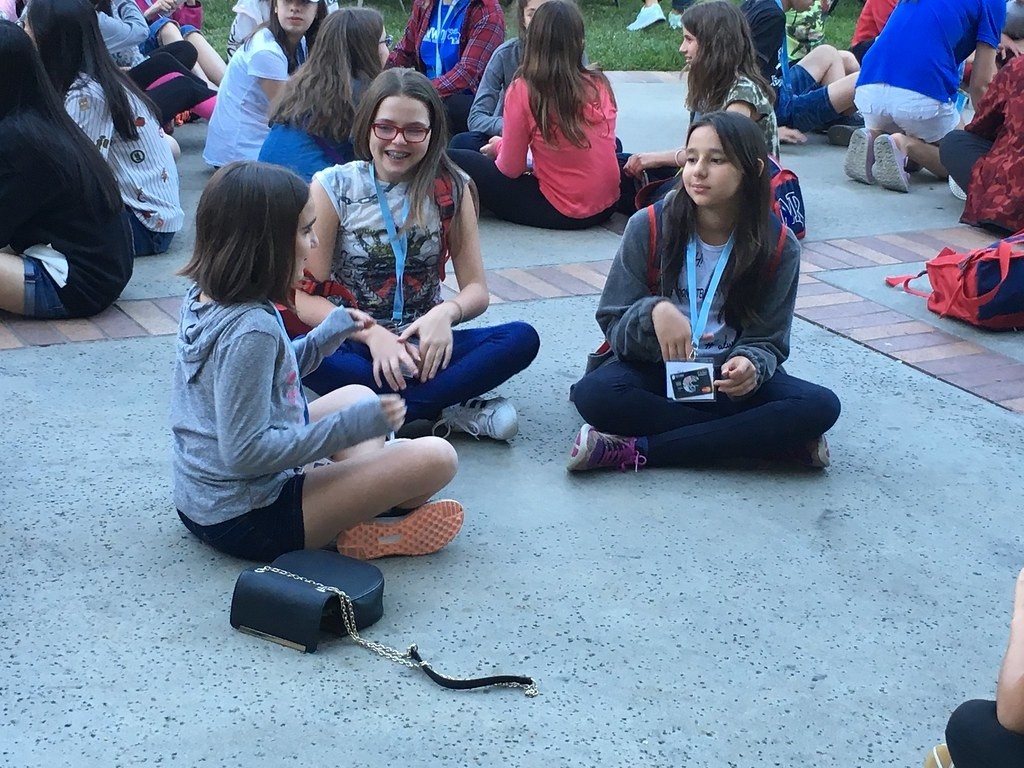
0 18 134 318
168 161 463 565
567 110 841 473
295 68 540 442
0 0 1024 255
926 568 1024 768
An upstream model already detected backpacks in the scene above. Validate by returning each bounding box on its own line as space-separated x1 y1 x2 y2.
767 150 807 243
885 228 1024 330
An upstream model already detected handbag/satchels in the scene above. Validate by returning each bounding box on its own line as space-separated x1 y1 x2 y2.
230 547 539 698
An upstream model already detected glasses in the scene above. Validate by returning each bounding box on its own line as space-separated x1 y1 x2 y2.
369 120 433 143
377 34 393 46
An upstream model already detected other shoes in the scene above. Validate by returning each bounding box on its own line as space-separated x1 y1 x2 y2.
337 498 464 560
164 111 201 135
949 174 967 201
828 123 865 146
815 108 865 134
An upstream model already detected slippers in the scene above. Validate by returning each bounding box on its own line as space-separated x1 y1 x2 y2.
871 134 911 193
844 128 877 187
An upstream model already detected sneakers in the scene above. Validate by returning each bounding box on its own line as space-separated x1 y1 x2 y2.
432 390 519 441
778 433 830 470
669 11 682 30
566 422 648 473
626 4 666 31
